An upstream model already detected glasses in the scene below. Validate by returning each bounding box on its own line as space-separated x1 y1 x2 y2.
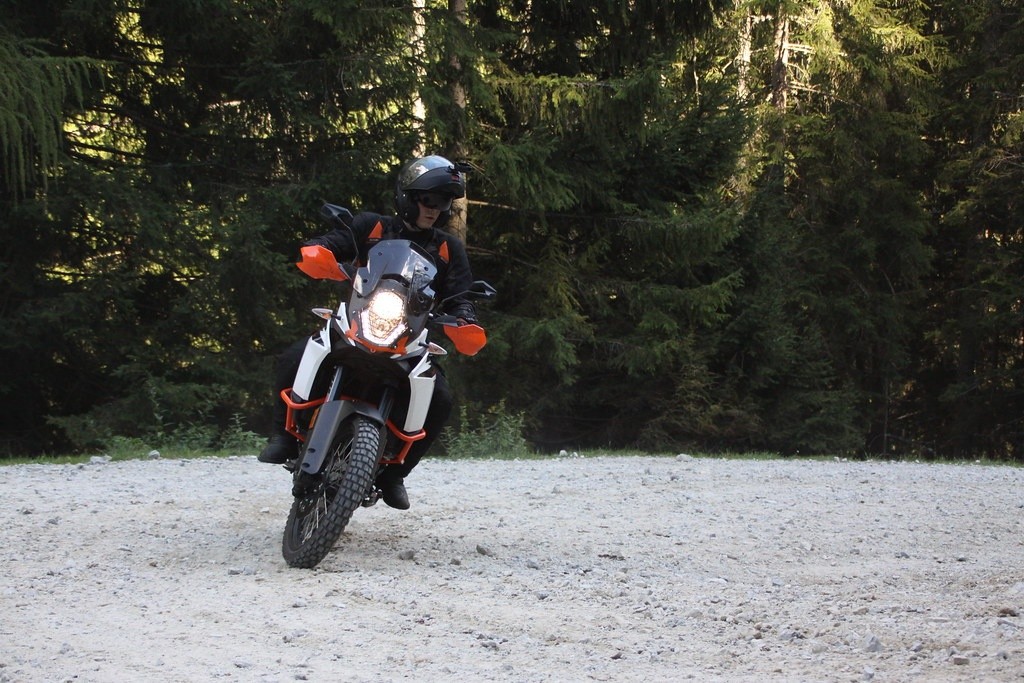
416 189 454 211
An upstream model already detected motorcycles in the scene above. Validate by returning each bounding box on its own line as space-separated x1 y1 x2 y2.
260 200 498 571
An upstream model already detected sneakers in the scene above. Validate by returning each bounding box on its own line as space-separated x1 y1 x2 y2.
258 429 298 463
374 466 410 509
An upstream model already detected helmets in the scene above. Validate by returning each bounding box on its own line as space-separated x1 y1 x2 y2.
393 155 471 228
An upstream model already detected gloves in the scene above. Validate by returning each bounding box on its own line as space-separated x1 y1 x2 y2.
306 239 330 250
453 304 479 326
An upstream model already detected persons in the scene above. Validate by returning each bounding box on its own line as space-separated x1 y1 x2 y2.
257 155 479 510
